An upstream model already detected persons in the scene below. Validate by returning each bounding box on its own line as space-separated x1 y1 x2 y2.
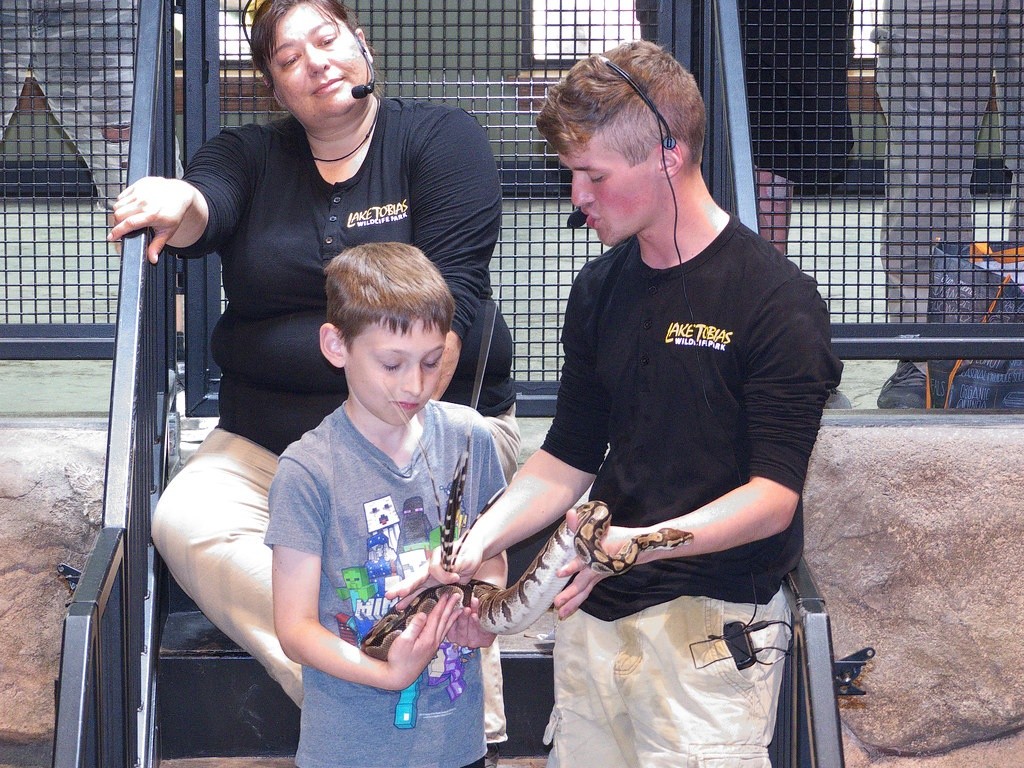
264 239 512 767
869 1 1023 410
384 40 843 767
105 0 509 766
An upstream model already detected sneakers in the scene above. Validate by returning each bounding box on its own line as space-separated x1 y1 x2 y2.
878 363 930 409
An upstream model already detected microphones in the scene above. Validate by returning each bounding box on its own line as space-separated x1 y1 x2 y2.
566 210 587 228
351 51 376 99
